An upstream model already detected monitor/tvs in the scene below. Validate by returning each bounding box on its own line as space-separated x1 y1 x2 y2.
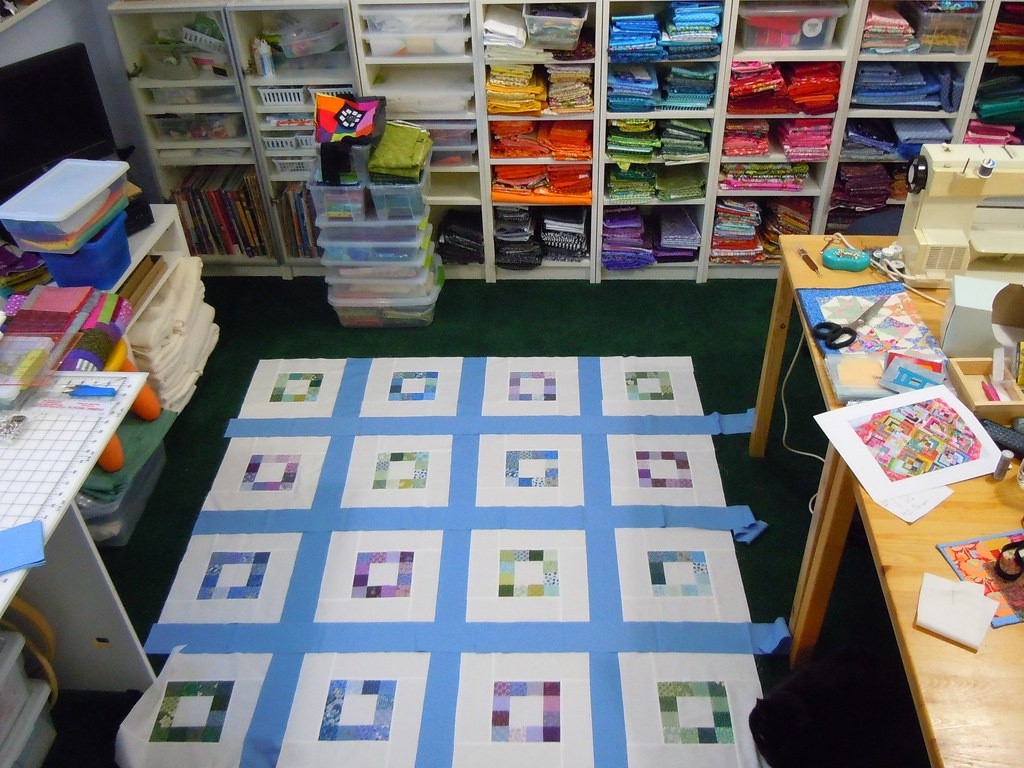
0 42 118 206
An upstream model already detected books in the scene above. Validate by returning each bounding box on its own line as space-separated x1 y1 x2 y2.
277 181 322 258
169 164 278 258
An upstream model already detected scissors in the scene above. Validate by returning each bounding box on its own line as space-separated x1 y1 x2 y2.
813 293 892 350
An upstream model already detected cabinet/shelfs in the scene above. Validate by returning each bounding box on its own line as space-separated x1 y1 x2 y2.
105 0 1001 284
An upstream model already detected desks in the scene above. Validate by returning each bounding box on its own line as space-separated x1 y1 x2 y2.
748 234 951 633
791 425 1024 768
0 205 192 692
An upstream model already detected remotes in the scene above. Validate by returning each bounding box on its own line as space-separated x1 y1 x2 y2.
981 419 1024 463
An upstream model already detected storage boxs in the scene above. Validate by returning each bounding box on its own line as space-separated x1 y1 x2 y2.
275 44 350 73
75 438 167 545
363 25 472 56
150 87 240 104
277 24 347 59
940 274 1009 359
948 356 1024 425
304 148 444 327
40 210 131 291
905 1 982 54
739 1 849 51
0 631 33 748
0 679 58 768
0 159 130 254
148 113 244 138
522 4 589 50
359 6 470 31
